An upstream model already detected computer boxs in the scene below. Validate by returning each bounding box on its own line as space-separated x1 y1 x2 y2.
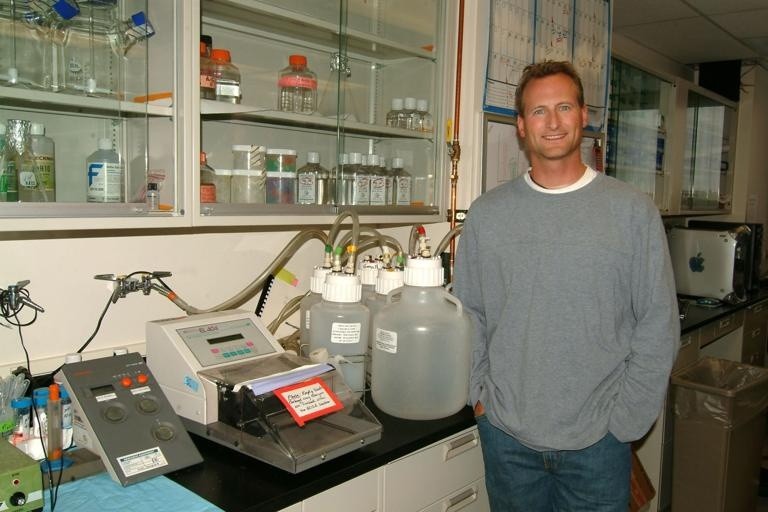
687 219 763 291
666 225 752 304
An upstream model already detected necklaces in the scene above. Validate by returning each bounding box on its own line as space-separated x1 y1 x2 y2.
529 173 578 189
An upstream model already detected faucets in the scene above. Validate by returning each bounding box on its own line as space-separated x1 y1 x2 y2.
9 280 45 312
94 270 174 303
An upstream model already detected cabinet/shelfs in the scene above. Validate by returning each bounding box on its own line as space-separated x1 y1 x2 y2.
608 53 740 216
666 301 766 365
382 421 495 512
195 0 452 224
0 1 196 235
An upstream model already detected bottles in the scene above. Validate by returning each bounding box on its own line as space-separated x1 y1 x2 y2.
19 124 56 203
85 138 125 204
231 144 266 205
145 183 159 210
200 41 242 104
200 152 216 203
277 55 318 116
10 383 73 461
266 148 297 204
329 151 413 207
386 97 433 134
297 151 329 205
1 124 19 202
216 169 231 204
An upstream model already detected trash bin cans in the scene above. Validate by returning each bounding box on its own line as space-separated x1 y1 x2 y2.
671 356 768 512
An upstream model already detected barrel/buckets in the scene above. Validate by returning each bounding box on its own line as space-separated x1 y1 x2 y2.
369 256 470 421
301 257 369 401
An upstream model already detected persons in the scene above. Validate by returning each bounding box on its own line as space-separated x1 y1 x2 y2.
449 59 682 512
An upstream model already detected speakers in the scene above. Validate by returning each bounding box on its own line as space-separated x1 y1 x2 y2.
698 59 741 101
